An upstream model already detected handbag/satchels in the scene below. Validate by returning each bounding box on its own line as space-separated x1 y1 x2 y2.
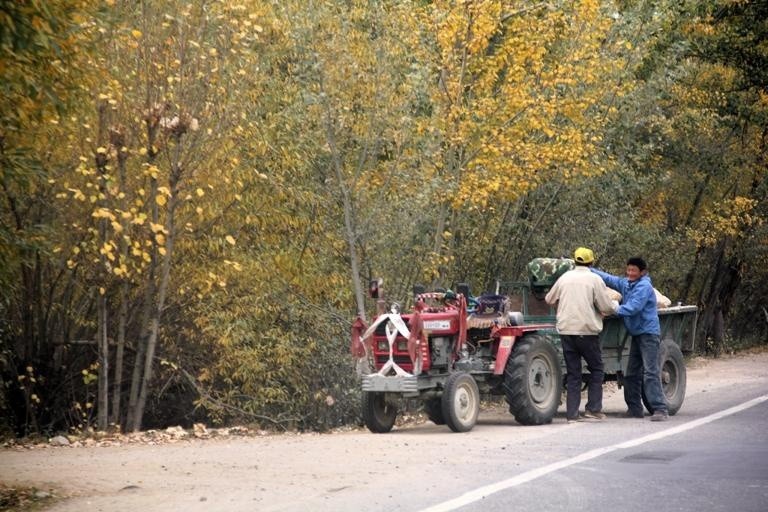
527 258 574 290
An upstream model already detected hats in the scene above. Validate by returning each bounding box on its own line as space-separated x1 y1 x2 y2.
575 246 594 264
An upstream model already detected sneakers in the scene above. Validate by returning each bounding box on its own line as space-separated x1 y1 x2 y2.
619 406 646 418
650 410 671 422
567 411 580 419
586 407 607 419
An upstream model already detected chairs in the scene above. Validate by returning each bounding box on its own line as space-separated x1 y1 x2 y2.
466 294 509 342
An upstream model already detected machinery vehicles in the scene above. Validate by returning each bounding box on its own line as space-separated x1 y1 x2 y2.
353 271 699 434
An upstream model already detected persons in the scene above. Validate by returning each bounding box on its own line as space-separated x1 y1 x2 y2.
542 247 619 425
588 256 669 421
